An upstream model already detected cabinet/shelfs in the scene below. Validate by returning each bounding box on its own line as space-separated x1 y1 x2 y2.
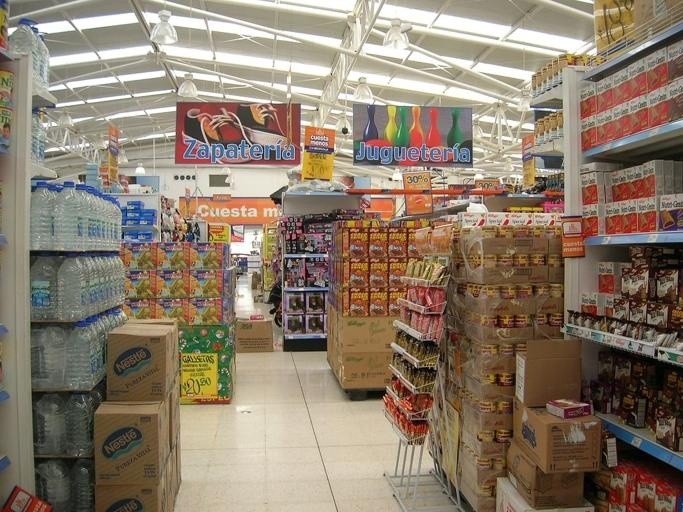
108 192 209 247
526 18 681 510
2 44 107 512
276 195 368 348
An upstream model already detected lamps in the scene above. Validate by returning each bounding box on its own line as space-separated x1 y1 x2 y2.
136 140 145 175
53 64 72 128
118 119 128 165
